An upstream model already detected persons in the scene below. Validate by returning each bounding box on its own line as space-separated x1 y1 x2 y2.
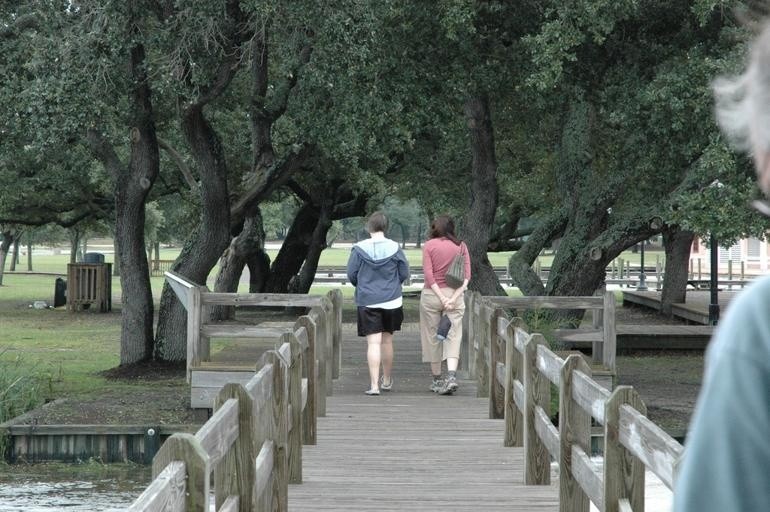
666 0 770 511
420 216 471 395
346 212 410 397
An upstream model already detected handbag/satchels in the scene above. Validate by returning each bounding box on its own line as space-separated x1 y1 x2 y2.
445 255 465 288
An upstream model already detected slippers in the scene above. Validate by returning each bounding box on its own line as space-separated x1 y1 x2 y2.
380 376 393 390
366 389 380 395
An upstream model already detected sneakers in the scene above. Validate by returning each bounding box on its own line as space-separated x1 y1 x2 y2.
430 375 458 395
